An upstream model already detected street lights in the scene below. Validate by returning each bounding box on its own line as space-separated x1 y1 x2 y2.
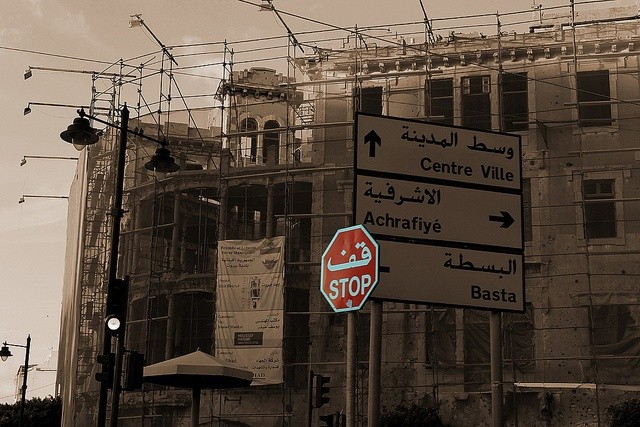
59 103 181 427
0 335 31 421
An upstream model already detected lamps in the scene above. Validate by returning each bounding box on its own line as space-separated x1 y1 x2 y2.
24 103 31 115
20 156 27 166
19 195 25 204
128 13 142 28
260 0 274 12
24 67 32 80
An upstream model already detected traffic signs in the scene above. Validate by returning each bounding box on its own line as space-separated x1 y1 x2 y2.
321 225 380 314
354 110 526 313
